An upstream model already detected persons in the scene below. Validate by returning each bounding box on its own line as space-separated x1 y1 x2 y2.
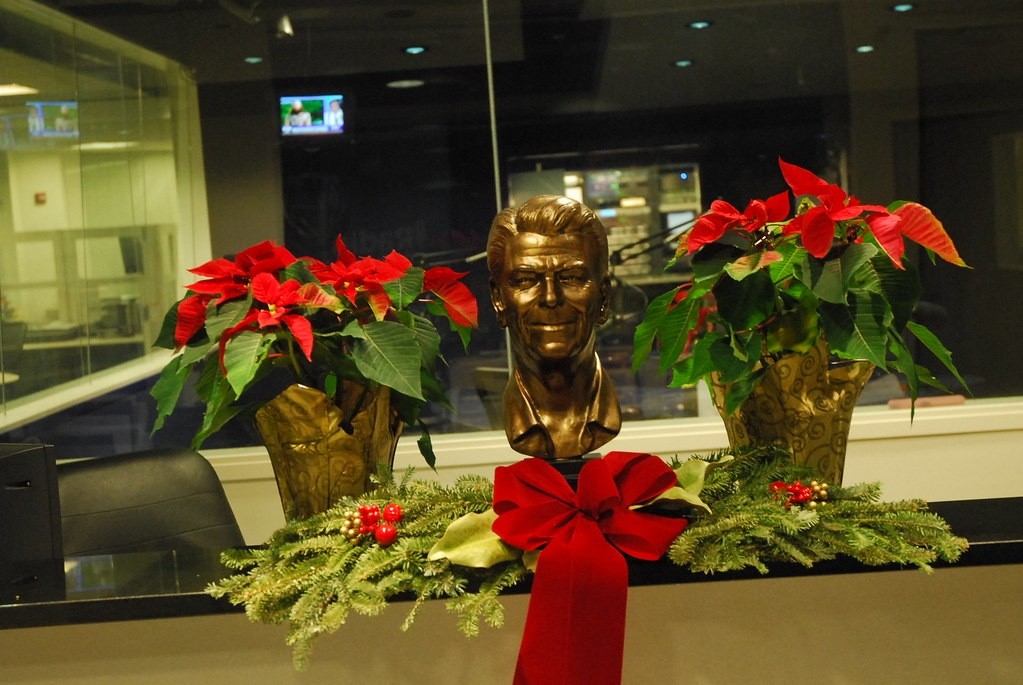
487 194 623 459
285 99 344 131
27 106 76 134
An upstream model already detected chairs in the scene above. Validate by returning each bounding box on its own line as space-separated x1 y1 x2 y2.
54 445 259 599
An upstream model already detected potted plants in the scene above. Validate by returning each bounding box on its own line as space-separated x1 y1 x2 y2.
145 233 483 522
628 153 973 485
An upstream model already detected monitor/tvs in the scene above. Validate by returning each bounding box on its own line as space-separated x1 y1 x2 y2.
277 90 354 144
23 99 81 140
